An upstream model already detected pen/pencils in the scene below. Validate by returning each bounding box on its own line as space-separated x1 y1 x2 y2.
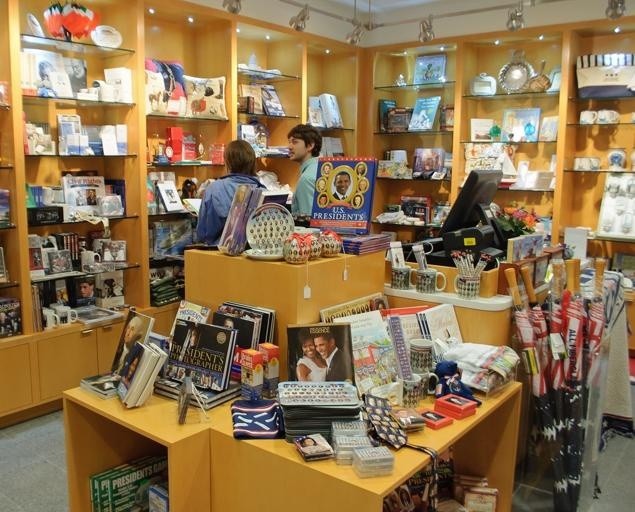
451 250 491 277
411 244 428 273
387 239 406 271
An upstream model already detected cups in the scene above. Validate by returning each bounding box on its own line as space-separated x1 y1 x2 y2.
454 274 482 300
409 340 433 371
55 306 78 325
417 269 446 293
41 309 60 329
596 110 619 124
579 111 597 124
80 251 101 270
389 263 415 288
417 370 439 395
401 373 421 408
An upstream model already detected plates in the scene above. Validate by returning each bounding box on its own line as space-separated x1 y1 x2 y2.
243 248 283 260
245 202 295 249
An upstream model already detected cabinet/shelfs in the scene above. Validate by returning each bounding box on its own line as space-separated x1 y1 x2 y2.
222 0 242 15
183 244 387 359
366 15 635 359
0 1 365 430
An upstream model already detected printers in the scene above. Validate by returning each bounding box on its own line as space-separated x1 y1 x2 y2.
386 228 506 272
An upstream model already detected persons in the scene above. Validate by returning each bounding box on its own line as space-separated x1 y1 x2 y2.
297 328 327 381
299 437 329 454
288 124 323 228
196 140 268 245
314 333 352 381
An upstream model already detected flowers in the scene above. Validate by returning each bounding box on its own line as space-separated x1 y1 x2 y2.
495 207 537 236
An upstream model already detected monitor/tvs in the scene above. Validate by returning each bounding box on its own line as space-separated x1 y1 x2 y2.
436 168 504 238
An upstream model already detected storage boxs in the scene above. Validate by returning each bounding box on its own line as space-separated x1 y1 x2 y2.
385 283 550 347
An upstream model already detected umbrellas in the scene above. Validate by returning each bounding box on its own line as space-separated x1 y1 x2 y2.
504 258 607 512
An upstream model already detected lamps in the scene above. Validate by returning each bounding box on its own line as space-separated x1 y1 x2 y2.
506 0 525 32
606 0 626 20
289 4 310 33
345 1 364 47
419 15 435 43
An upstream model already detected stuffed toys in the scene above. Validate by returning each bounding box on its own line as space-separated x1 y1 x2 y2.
435 360 482 406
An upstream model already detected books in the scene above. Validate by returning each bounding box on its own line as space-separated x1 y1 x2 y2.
287 323 354 386
507 236 527 263
310 157 391 255
33 269 124 333
377 148 453 180
257 191 290 209
27 170 126 226
319 136 344 158
378 96 454 133
0 246 10 283
380 304 463 380
564 227 587 260
238 123 290 154
154 300 277 409
21 115 128 156
520 233 544 258
21 47 133 103
239 82 285 116
332 310 400 399
0 297 22 338
384 445 498 512
413 53 447 89
320 293 390 324
308 94 344 128
80 307 168 409
29 230 127 278
0 189 11 229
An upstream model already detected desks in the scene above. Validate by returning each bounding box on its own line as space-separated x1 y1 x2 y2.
62 380 523 512
